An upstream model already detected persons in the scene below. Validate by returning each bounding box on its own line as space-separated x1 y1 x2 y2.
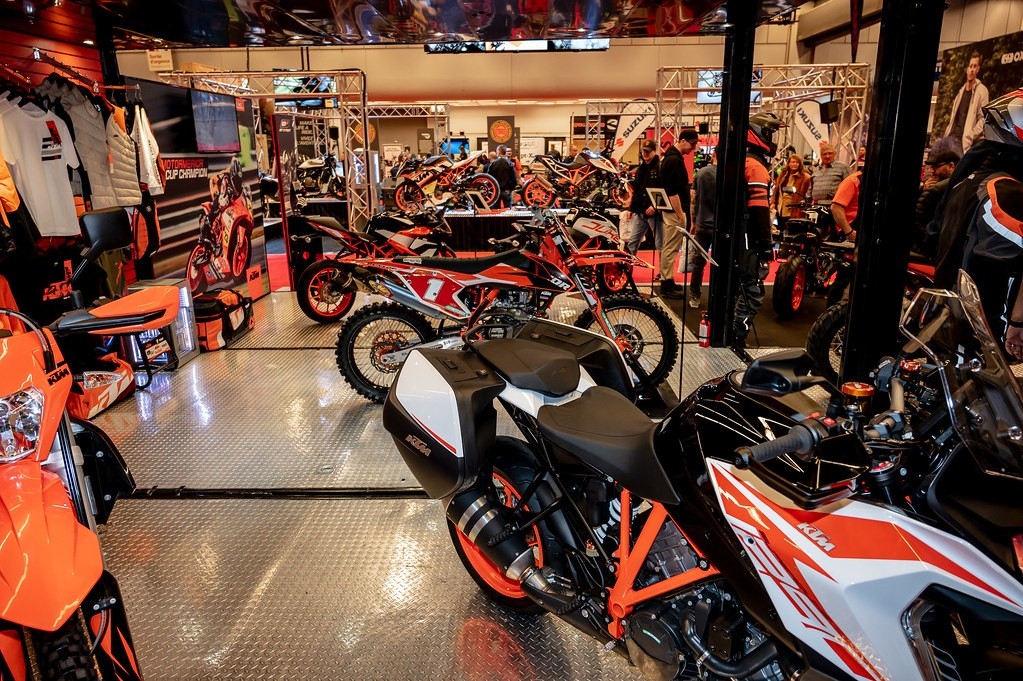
546 144 560 161
563 145 578 163
689 146 718 307
770 86 1023 360
483 151 497 173
457 146 467 160
943 52 989 154
659 130 698 299
488 145 517 209
627 140 663 282
505 146 516 173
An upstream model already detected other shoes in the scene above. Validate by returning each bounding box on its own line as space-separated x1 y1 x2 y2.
658 280 683 298
688 297 699 309
668 281 682 290
653 273 663 281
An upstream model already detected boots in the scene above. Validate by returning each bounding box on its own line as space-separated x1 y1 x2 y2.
732 321 753 365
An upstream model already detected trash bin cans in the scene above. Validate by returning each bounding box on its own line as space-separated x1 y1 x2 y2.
288 215 325 291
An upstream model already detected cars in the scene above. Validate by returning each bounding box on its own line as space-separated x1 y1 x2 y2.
520 155 554 177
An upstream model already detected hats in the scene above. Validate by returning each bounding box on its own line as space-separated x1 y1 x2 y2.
679 130 702 142
643 140 657 152
924 151 964 165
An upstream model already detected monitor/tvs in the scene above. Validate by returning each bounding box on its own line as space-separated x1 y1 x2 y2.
272 68 338 108
696 70 762 104
120 74 241 156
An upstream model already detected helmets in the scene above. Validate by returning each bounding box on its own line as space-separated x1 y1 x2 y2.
747 112 790 157
981 87 1023 145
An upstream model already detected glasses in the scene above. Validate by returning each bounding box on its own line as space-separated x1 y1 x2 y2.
685 139 697 146
931 162 948 173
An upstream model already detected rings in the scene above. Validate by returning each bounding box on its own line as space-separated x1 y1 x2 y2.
1012 345 1014 347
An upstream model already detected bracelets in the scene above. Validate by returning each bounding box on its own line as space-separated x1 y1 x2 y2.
1010 319 1023 328
845 230 853 236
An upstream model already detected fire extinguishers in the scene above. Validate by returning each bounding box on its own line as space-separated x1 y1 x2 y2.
699 310 711 348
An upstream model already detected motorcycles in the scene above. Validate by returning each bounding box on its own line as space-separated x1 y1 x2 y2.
184 183 255 296
390 151 500 211
770 186 837 313
296 198 456 323
382 269 1023 681
803 242 936 390
334 195 678 405
519 137 635 211
297 144 347 200
0 206 181 681
